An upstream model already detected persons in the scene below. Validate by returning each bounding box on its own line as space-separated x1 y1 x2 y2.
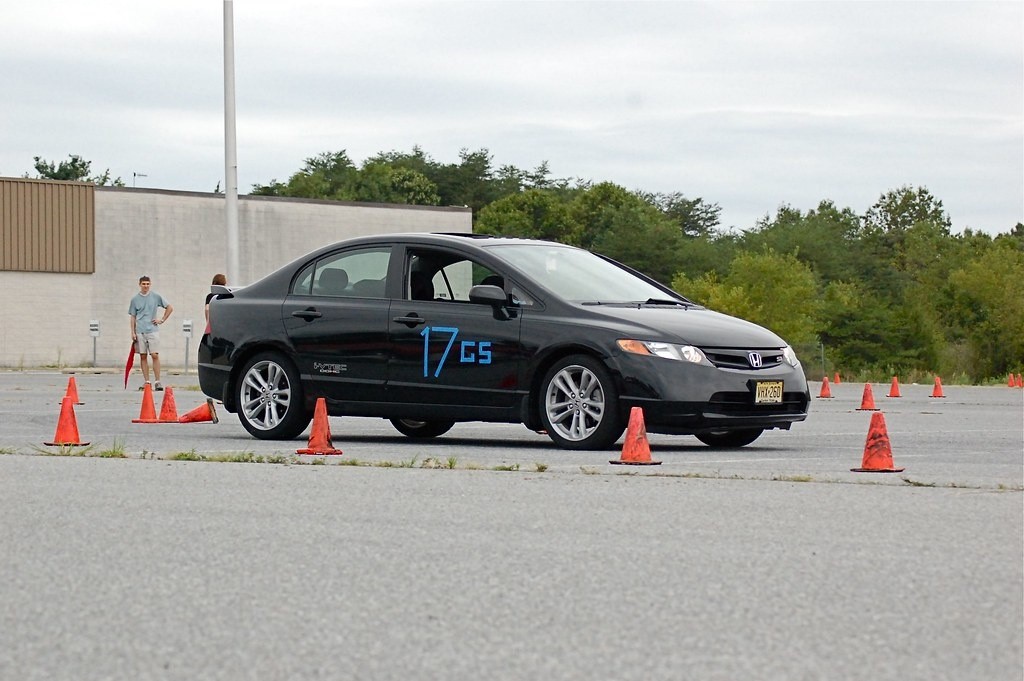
205 274 227 404
128 276 173 391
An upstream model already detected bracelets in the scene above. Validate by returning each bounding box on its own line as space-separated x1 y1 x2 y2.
161 319 164 322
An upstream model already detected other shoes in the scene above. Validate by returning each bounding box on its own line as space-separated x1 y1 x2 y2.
155 381 163 391
139 383 152 390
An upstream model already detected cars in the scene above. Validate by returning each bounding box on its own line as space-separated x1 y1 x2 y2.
197 230 811 452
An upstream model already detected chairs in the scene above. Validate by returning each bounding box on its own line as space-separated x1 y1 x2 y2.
481 274 505 291
409 270 435 301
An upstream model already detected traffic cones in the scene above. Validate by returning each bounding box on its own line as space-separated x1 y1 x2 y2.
834 373 841 384
816 377 835 398
929 378 946 398
296 397 343 455
609 406 662 466
855 382 880 411
158 386 180 423
849 413 906 474
1008 374 1024 387
886 376 902 397
179 398 219 424
59 377 85 405
44 396 91 447
132 382 160 424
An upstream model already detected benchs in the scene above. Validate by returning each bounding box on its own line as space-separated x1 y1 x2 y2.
312 269 386 297
